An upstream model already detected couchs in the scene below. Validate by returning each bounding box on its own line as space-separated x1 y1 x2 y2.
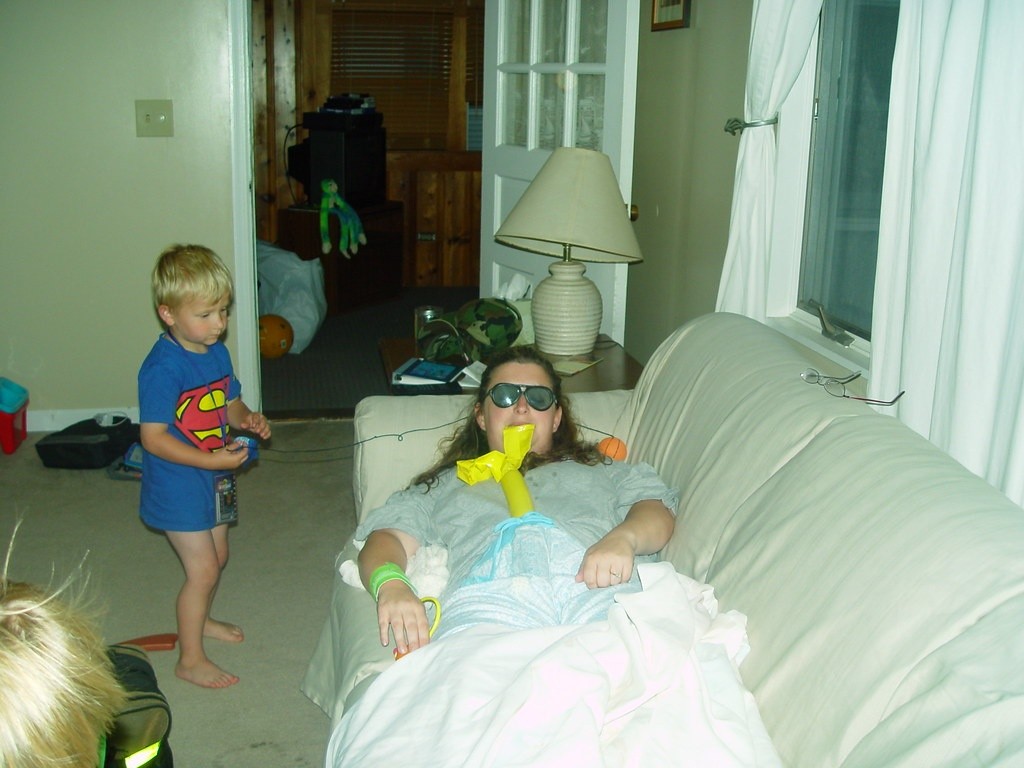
299 312 1024 768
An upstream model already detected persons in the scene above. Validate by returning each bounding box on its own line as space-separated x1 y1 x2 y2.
0 518 127 768
357 343 680 656
138 244 272 688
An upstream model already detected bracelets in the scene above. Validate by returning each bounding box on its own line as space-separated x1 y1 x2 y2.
367 564 418 602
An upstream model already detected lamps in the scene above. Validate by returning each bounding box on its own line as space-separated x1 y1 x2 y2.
493 147 643 354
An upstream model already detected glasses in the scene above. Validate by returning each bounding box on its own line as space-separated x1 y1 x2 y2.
482 382 558 412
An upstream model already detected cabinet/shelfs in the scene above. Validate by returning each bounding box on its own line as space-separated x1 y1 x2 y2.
280 200 405 314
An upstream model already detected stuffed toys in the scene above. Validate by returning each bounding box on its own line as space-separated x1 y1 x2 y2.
320 180 367 259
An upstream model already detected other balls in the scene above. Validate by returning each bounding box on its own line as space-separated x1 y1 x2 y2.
259 315 295 361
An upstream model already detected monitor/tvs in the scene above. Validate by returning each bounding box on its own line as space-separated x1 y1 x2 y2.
287 128 387 207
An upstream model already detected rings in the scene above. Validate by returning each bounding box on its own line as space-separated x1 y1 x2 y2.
609 572 623 578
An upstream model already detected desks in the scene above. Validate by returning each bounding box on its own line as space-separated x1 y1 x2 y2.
378 334 644 396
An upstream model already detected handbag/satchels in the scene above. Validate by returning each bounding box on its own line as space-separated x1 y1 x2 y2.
413 297 524 368
102 643 174 768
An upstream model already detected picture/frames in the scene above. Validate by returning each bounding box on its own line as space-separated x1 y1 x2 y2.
651 0 691 32
